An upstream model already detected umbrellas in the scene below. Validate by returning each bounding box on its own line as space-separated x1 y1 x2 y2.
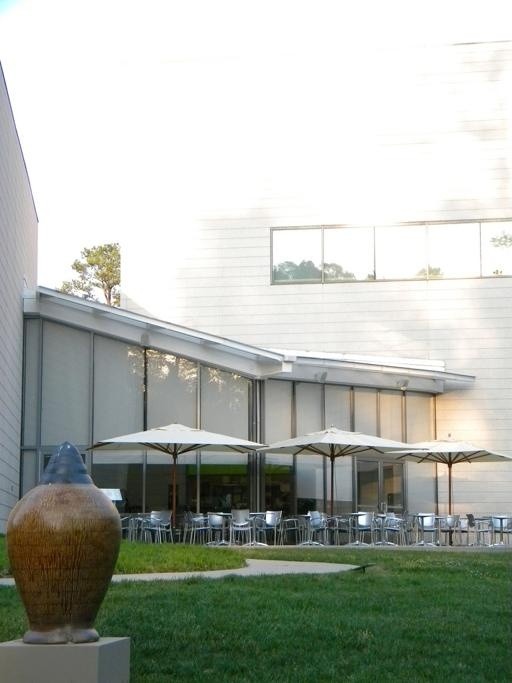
256 425 427 543
83 420 269 536
385 433 510 546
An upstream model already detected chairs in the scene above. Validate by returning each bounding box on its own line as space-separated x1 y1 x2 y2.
120 508 512 549
